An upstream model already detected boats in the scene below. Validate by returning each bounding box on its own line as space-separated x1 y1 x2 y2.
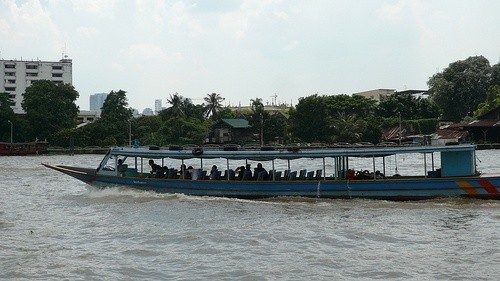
43 143 500 201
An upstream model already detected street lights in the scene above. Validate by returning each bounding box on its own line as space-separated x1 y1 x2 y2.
8 120 13 156
259 113 264 146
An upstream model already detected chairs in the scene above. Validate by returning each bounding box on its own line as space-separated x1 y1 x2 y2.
187 168 322 179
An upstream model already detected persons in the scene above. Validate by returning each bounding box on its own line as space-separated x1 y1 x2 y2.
185 166 194 180
254 163 265 180
151 165 164 178
149 160 157 178
235 164 252 181
209 165 218 180
174 164 189 179
118 159 128 172
163 166 173 178
